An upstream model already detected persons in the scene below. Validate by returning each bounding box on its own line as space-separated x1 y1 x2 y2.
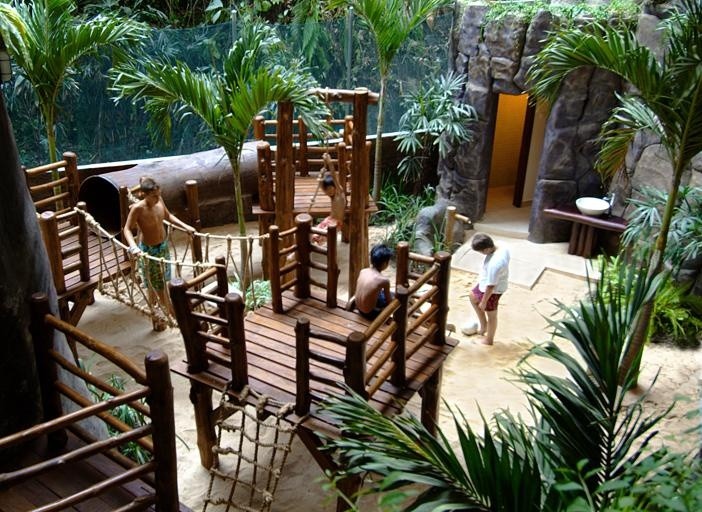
469 232 511 346
123 178 196 317
311 152 347 245
355 245 395 323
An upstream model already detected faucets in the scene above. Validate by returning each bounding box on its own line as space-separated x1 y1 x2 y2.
602 192 616 209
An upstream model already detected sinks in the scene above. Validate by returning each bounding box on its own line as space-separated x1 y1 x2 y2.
576 197 610 216
